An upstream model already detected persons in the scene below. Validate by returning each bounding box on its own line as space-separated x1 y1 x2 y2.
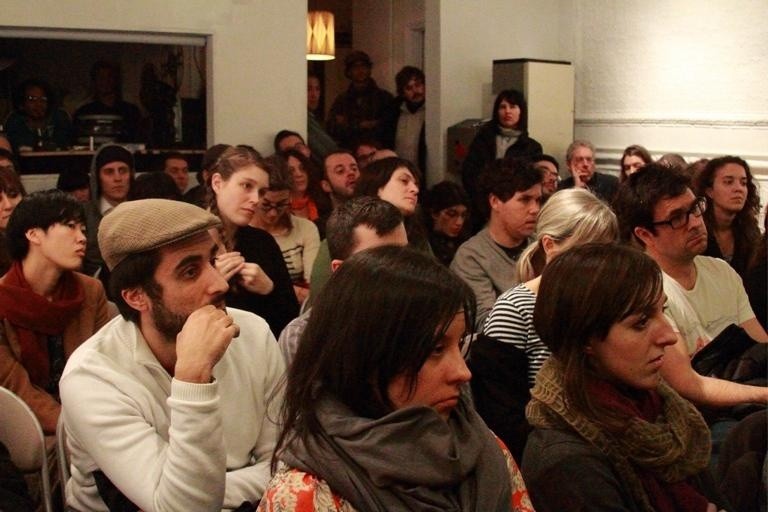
4 79 72 149
457 89 544 190
325 48 396 153
0 129 767 512
136 62 207 148
62 57 143 145
307 73 341 159
365 64 428 178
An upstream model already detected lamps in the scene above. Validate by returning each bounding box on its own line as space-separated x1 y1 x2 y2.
306 12 336 63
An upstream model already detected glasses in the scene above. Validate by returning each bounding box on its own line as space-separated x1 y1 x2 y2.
542 168 562 183
641 198 706 228
357 151 375 159
260 200 290 212
26 96 47 102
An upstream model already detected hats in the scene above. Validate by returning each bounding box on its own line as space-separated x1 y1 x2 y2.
96 144 133 172
96 198 221 272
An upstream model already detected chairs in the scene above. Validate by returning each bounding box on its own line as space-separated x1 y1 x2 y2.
0 388 51 510
56 409 76 511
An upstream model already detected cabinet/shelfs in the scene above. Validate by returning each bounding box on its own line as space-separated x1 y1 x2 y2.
490 56 576 183
